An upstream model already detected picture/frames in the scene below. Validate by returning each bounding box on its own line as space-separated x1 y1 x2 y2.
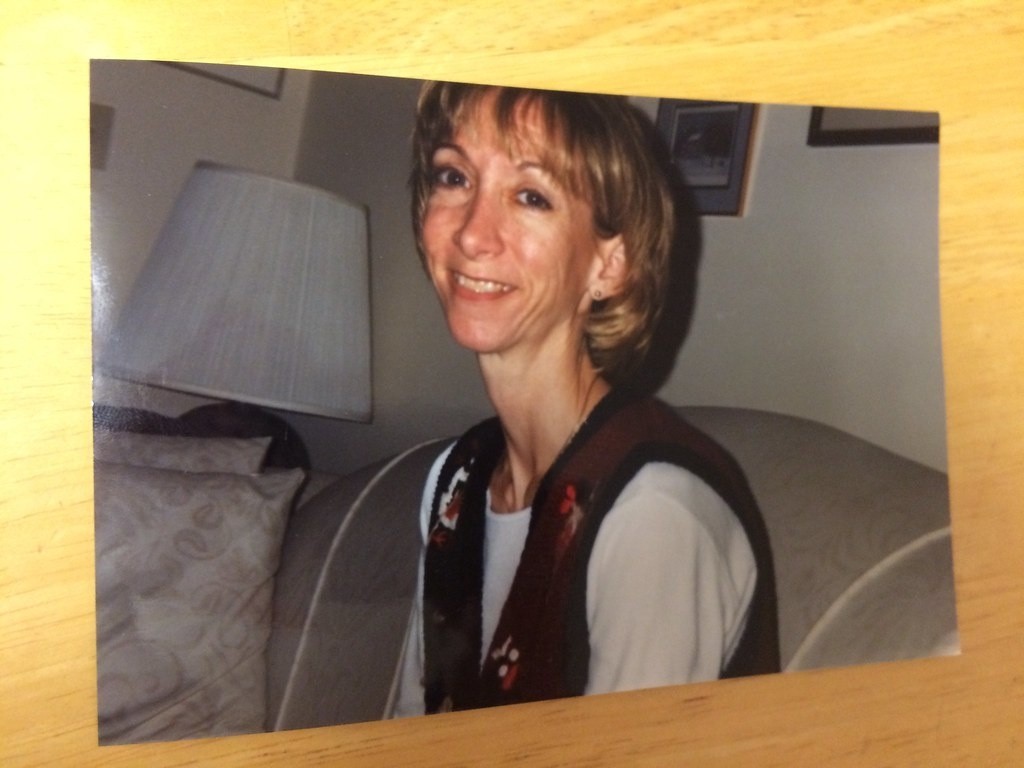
649 97 756 217
804 107 939 148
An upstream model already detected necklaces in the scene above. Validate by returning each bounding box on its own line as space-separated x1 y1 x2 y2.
484 367 602 515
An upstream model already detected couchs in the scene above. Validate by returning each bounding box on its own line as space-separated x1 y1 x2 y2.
92 404 951 744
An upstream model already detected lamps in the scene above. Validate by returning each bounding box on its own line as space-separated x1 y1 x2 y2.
93 162 371 482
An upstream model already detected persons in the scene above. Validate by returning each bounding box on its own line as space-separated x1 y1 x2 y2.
376 76 781 722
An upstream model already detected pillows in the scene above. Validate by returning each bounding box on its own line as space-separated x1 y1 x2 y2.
86 421 308 742
